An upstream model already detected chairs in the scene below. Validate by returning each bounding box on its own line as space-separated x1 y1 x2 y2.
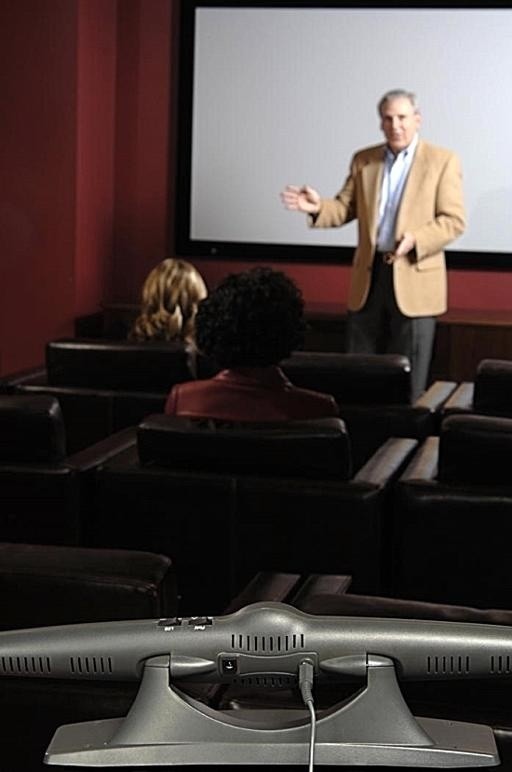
0 332 512 728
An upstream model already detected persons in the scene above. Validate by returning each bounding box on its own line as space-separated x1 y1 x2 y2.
164 265 341 423
281 89 467 403
128 258 209 352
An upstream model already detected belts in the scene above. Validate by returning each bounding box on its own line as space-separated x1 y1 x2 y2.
375 252 395 266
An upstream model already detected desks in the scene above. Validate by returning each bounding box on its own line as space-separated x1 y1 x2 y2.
74 303 509 382
